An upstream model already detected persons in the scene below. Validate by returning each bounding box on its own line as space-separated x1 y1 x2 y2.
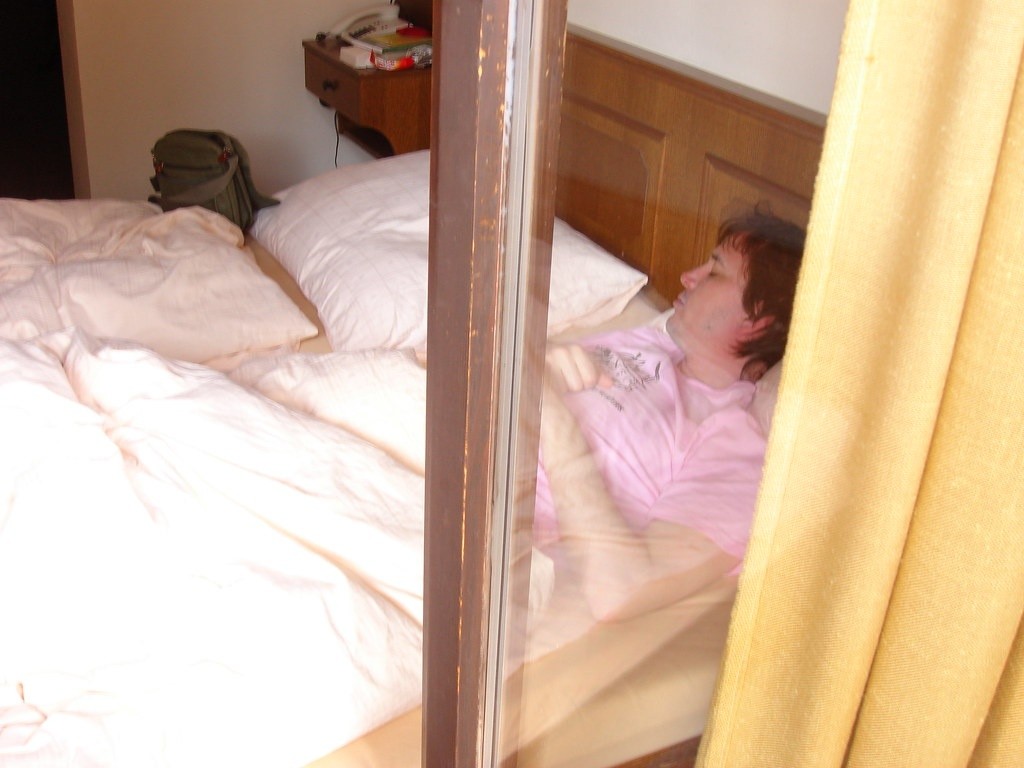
533 205 806 625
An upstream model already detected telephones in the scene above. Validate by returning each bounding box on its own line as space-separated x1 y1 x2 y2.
329 5 411 45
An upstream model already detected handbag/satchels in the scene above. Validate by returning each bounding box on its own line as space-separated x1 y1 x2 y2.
149 127 280 233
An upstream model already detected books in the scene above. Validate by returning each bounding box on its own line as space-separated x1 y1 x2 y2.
339 30 433 71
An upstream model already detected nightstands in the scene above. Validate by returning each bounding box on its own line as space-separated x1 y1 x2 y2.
302 35 433 161
